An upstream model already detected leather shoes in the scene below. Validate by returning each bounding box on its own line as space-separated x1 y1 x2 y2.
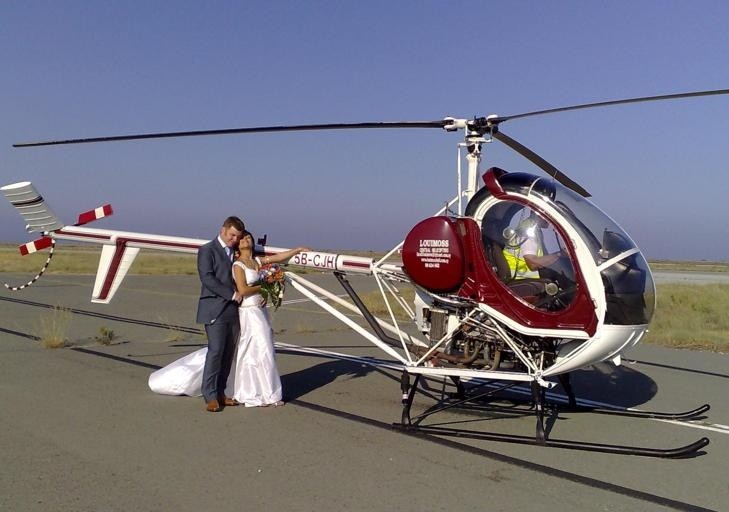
221 398 239 406
206 399 220 412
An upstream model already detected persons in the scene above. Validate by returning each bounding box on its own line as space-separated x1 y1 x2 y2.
195 215 245 413
148 230 309 407
503 209 569 280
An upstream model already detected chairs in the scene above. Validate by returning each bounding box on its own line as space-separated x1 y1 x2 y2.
492 224 546 297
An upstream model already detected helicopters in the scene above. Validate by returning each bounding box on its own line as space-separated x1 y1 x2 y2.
1 88 715 458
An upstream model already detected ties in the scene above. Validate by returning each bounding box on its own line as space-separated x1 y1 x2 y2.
226 248 233 260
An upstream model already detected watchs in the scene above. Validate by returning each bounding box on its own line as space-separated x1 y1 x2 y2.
555 252 563 258
